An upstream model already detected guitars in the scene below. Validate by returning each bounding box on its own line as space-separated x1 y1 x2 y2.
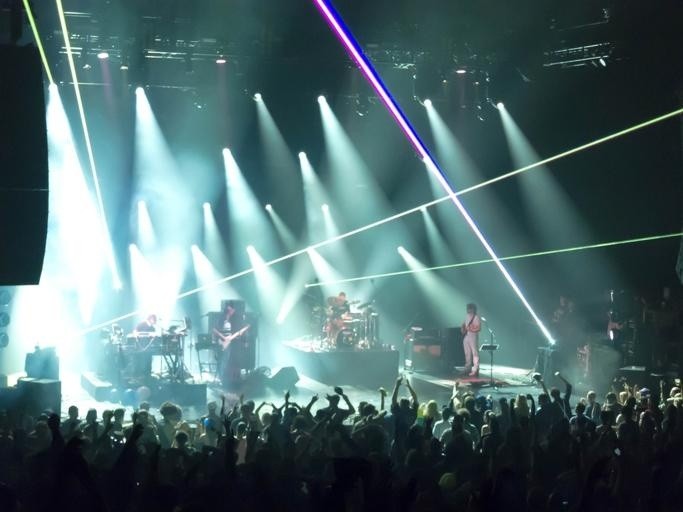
218 325 252 351
460 315 477 344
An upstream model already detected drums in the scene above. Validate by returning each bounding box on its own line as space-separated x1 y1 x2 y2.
336 328 357 351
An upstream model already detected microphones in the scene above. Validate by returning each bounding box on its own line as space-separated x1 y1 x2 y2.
481 316 487 323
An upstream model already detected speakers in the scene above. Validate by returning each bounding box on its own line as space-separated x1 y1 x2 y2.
1 43 48 286
26 346 59 380
18 377 61 424
618 365 647 390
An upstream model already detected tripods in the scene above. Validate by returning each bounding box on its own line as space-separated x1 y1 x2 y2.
481 351 505 391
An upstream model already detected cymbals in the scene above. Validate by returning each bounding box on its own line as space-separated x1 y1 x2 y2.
358 303 371 309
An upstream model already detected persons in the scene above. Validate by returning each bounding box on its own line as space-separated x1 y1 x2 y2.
549 284 683 377
0 378 682 512
327 292 348 341
135 315 157 332
459 303 482 379
213 300 247 378
